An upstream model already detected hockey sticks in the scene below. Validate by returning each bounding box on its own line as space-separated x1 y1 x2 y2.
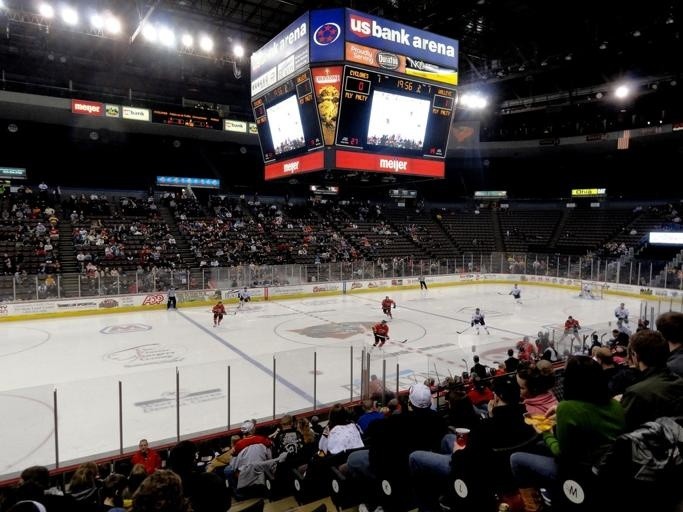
457 326 471 334
211 312 237 315
497 293 514 295
375 334 408 343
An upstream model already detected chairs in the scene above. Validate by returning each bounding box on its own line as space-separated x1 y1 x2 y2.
0 192 683 302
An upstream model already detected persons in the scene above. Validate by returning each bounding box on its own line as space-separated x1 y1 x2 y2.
210 350 535 512
367 93 423 152
0 439 232 511
238 287 251 308
372 320 389 349
212 301 227 327
509 311 683 511
268 107 304 155
166 286 176 310
471 308 489 335
382 296 396 319
615 303 629 329
1 181 683 299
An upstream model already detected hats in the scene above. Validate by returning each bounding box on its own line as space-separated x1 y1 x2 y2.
241 423 254 432
408 384 432 407
484 375 520 400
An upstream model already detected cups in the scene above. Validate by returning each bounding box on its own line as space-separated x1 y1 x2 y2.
454 428 470 448
